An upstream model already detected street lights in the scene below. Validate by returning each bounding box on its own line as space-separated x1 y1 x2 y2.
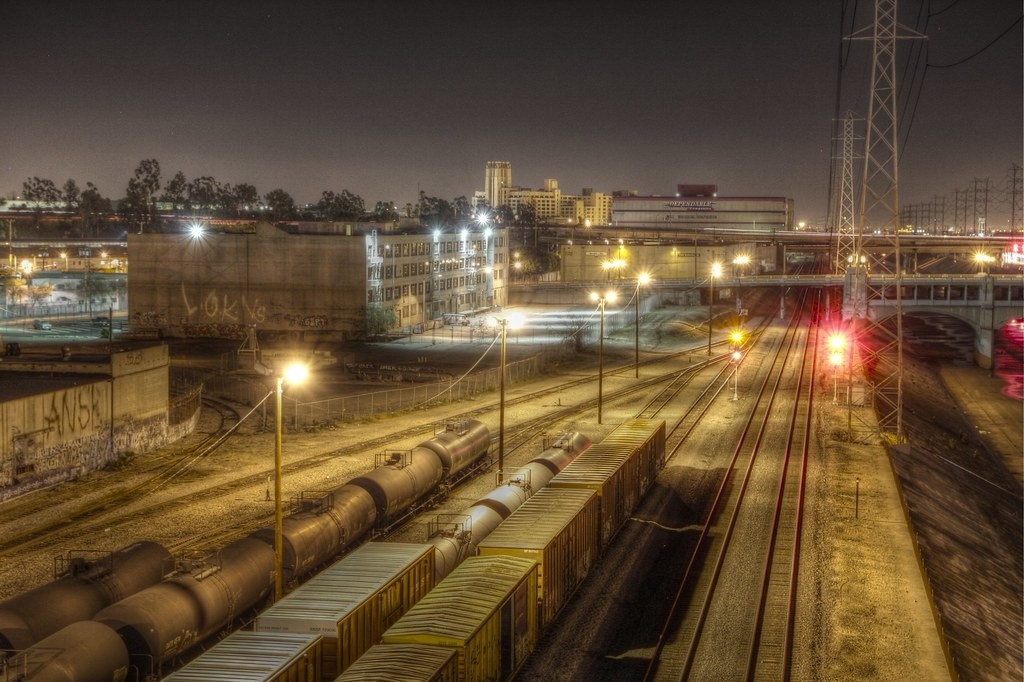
972 250 998 376
731 329 743 401
736 253 751 328
636 272 656 378
589 288 611 423
61 253 66 271
272 360 315 604
707 261 724 356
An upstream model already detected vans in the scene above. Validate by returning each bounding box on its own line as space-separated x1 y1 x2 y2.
444 314 470 327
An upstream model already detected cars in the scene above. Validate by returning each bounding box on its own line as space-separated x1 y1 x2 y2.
34 321 53 331
93 317 110 327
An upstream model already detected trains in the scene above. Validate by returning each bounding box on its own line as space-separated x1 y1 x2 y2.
315 416 674 682
0 418 490 682
1 540 178 668
151 429 587 681
